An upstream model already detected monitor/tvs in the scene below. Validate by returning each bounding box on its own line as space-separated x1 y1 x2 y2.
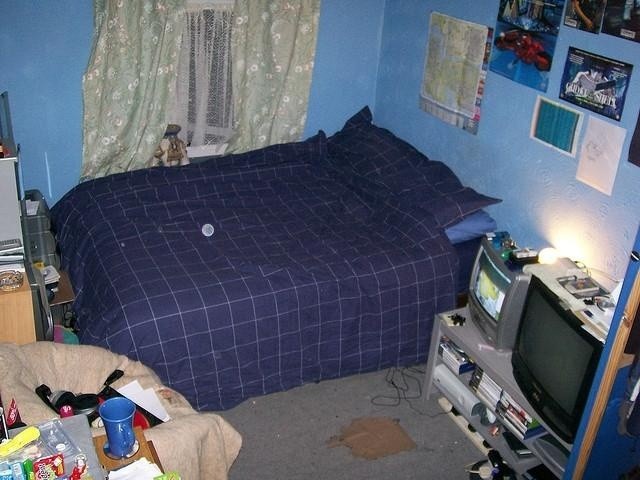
469 237 532 351
511 267 610 454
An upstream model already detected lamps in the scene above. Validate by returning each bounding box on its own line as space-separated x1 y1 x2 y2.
538 247 561 264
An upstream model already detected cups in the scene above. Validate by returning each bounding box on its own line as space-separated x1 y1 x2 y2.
98 396 136 457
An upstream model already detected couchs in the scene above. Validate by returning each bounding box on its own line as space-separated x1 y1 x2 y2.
0 340 242 480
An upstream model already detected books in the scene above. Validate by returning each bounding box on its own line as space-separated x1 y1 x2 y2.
469 366 547 441
439 336 476 375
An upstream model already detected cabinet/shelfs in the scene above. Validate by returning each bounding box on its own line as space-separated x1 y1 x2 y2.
423 306 575 479
1 144 26 262
0 263 54 343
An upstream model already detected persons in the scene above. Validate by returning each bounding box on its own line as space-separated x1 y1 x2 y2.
570 65 615 109
575 0 601 33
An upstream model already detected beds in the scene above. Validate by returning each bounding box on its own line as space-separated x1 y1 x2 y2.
47 106 517 413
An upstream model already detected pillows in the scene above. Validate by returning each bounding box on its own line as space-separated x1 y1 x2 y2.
327 106 428 189
380 160 503 228
